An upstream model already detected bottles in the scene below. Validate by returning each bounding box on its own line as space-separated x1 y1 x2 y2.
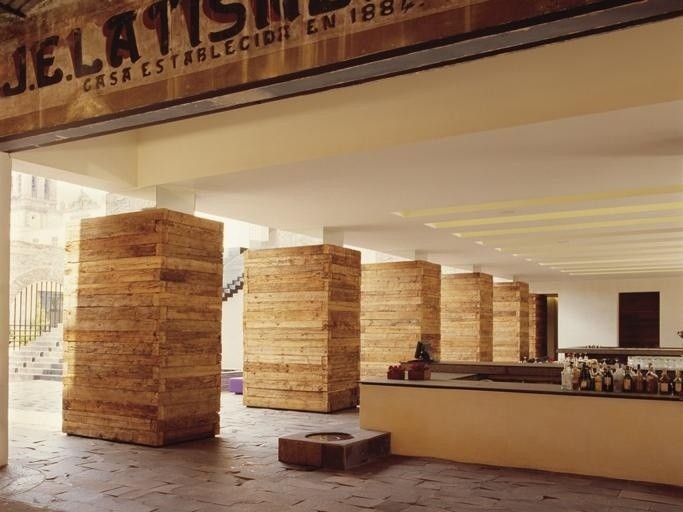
562 352 683 396
